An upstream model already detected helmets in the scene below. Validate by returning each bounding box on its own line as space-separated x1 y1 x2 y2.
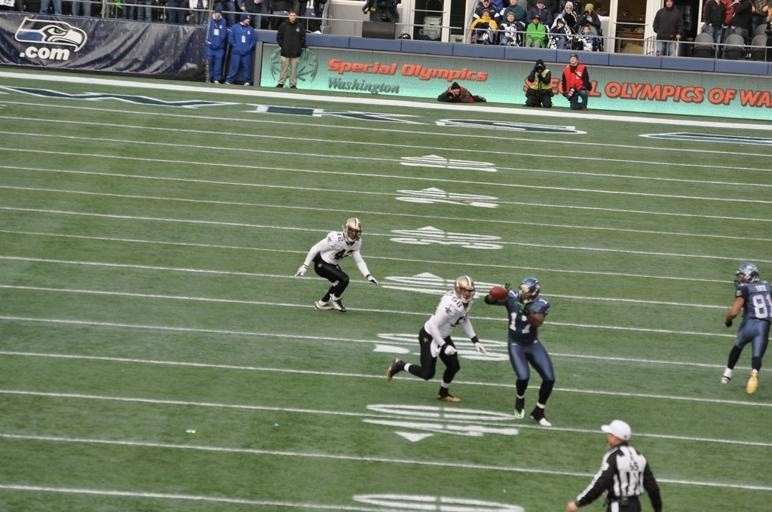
520 276 541 301
733 261 760 286
454 275 476 304
342 216 362 245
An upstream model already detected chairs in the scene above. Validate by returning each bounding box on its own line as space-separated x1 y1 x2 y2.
473 0 772 61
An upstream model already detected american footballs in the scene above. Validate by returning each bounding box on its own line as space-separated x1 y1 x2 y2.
489 287 507 299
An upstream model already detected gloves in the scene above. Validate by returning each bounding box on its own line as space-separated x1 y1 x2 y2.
443 345 458 356
294 263 310 278
365 273 379 287
475 342 487 357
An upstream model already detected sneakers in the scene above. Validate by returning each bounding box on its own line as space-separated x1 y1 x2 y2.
746 371 760 395
313 292 348 313
276 83 296 88
207 78 233 85
719 374 733 384
513 397 553 427
386 357 404 381
437 391 461 403
244 82 250 87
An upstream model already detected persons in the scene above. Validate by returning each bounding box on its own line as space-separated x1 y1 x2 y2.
437 81 487 104
275 10 307 90
720 262 772 396
562 54 592 109
564 416 665 512
225 13 260 87
482 276 557 428
523 59 553 108
387 272 486 402
203 5 228 85
295 216 381 314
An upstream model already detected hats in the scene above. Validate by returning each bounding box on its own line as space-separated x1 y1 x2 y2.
482 0 595 29
214 5 222 12
600 419 632 440
239 12 251 20
451 82 461 89
535 58 545 67
